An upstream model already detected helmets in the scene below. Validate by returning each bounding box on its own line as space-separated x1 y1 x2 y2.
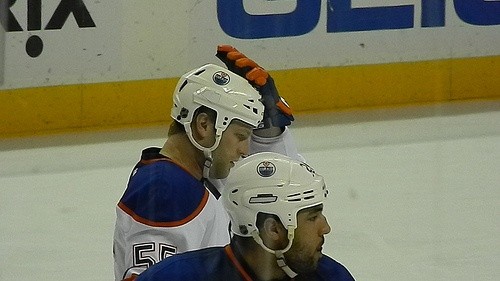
170 63 265 131
221 151 328 236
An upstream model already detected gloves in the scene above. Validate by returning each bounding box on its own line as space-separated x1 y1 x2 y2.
217 44 295 128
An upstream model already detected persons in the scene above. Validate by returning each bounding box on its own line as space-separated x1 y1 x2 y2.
111 43 307 281
137 151 355 281
